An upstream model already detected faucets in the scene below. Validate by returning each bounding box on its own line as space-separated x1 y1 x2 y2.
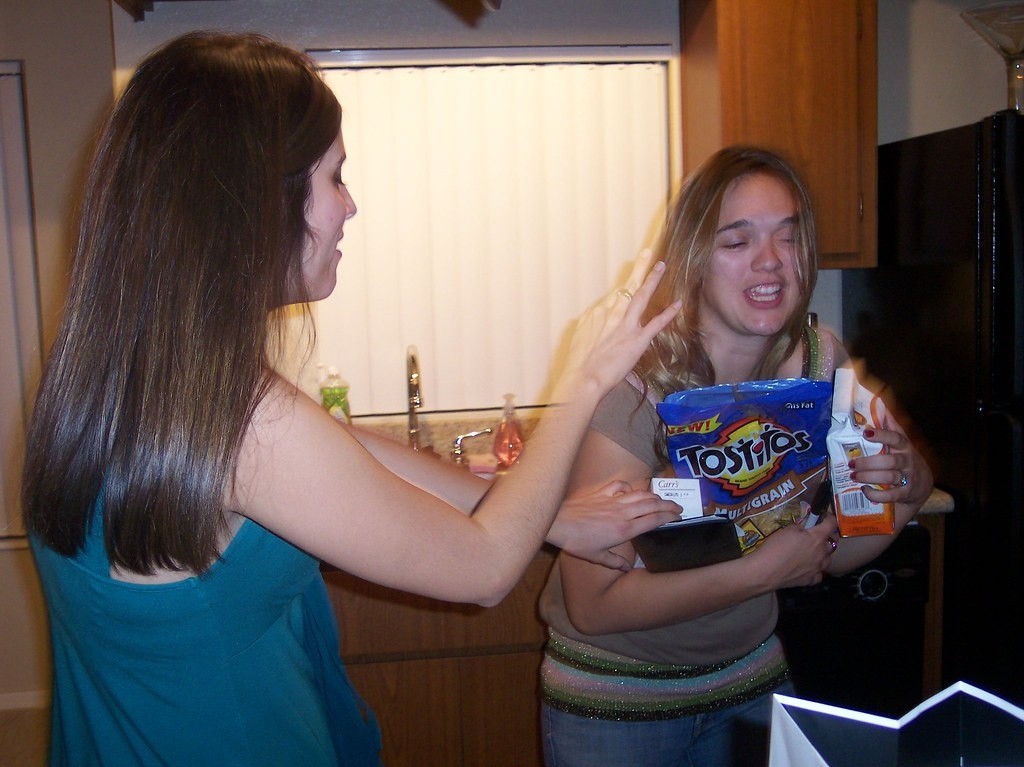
405 346 422 452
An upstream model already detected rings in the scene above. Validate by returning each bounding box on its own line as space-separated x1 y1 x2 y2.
892 468 907 488
616 290 633 300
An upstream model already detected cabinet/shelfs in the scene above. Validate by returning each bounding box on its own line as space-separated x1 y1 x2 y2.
0 0 117 766
843 111 1024 709
319 545 554 767
679 1 876 270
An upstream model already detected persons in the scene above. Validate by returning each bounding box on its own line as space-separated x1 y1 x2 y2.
544 132 930 762
19 28 697 767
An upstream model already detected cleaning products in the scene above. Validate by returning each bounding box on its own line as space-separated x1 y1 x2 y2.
829 369 895 539
657 380 834 555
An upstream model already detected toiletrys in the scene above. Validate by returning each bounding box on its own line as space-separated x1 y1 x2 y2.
493 390 523 464
318 366 352 425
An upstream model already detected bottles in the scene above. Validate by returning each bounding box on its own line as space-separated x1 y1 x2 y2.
319 368 352 428
